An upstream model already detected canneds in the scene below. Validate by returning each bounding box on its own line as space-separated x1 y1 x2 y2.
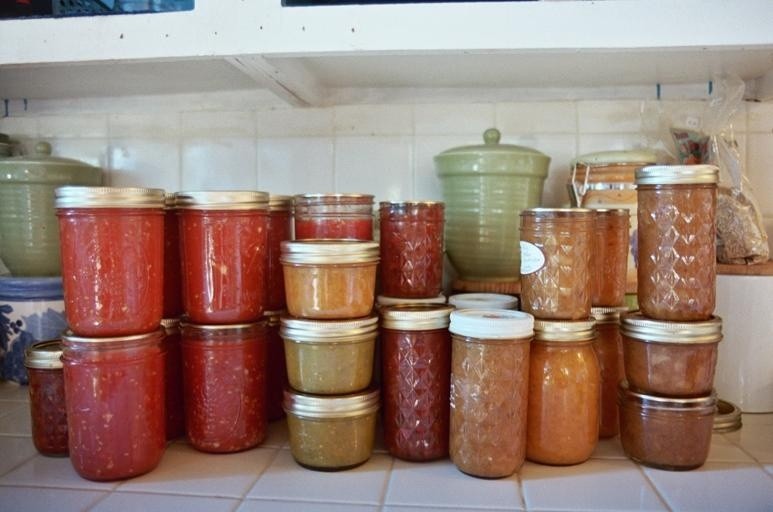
22 163 723 482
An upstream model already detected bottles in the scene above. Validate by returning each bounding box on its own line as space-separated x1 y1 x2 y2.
432 127 550 280
570 151 652 294
0 278 65 385
0 143 104 279
